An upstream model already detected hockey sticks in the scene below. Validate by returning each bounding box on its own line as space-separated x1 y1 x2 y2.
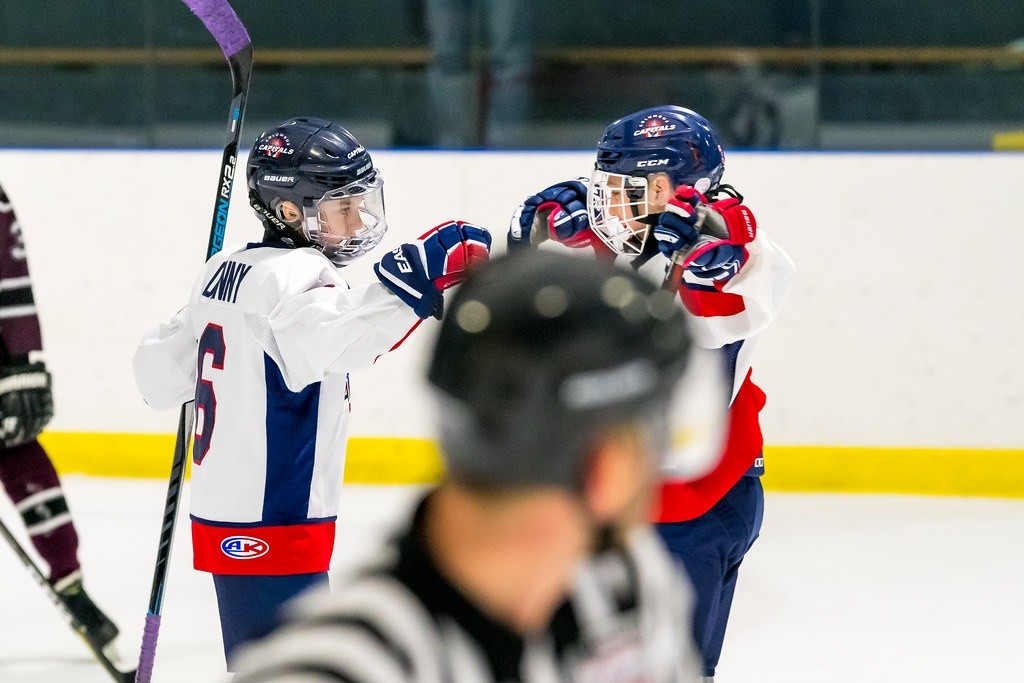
0 1 253 683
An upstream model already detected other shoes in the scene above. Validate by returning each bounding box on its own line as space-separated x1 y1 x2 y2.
47 564 122 660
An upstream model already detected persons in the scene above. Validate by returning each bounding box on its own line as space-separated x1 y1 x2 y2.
190 116 492 674
505 106 768 683
0 183 141 683
229 247 704 683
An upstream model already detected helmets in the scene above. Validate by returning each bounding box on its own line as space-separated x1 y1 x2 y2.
587 105 725 261
246 116 388 268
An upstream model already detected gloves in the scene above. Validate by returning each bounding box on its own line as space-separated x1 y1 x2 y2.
507 176 604 256
653 183 745 293
373 220 492 321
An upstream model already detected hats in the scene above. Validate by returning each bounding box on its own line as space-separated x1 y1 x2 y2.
424 248 694 422
